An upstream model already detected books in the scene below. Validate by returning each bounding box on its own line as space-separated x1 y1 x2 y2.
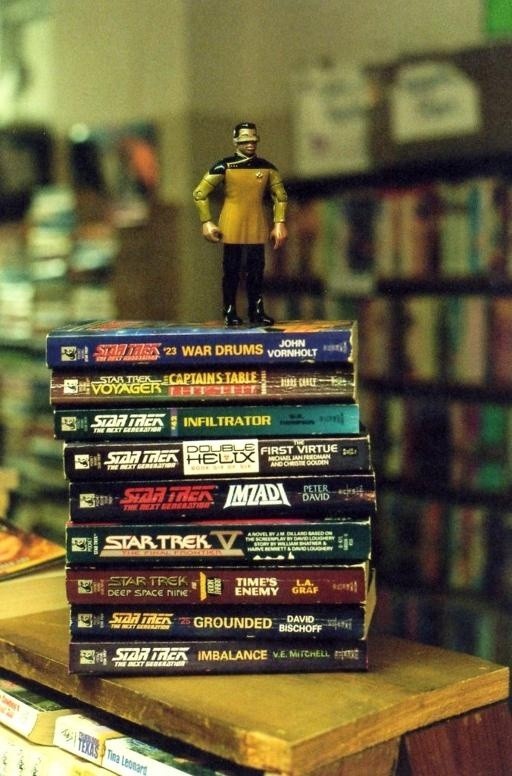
281 178 512 658
1 679 236 775
1 184 121 579
46 321 375 672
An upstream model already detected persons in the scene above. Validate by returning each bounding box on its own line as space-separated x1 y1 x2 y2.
193 123 288 326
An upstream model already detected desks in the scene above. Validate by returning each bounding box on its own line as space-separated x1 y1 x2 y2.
0 564 512 775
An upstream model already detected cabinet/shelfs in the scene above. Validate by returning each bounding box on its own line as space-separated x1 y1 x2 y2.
256 40 511 665
0 205 175 555
